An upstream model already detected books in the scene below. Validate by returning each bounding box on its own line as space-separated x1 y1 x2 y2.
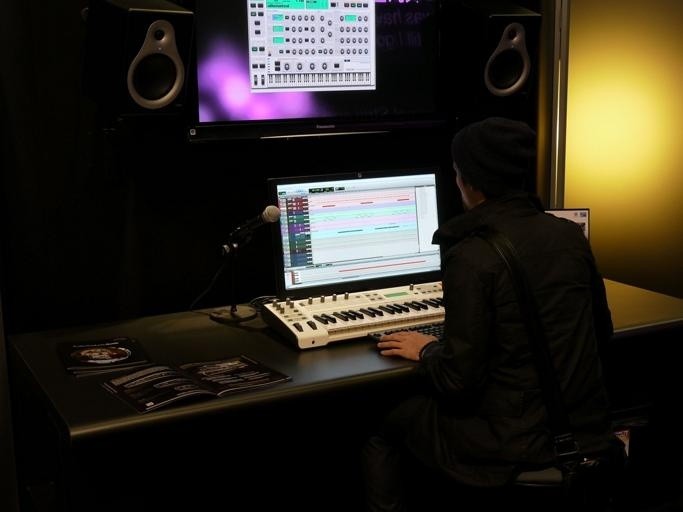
96 352 293 415
56 336 157 378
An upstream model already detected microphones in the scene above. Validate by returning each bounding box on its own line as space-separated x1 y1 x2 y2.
226 203 281 243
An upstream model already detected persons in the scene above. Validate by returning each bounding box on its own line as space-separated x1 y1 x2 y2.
363 117 614 512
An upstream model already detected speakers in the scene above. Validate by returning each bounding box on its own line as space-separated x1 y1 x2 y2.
478 4 543 129
109 0 201 134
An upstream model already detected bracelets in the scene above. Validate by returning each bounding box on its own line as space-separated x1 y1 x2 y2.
419 341 437 360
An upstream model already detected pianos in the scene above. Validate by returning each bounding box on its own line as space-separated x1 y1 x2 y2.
261 281 446 351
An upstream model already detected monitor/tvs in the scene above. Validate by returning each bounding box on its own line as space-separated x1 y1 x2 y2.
266 164 442 299
197 0 480 133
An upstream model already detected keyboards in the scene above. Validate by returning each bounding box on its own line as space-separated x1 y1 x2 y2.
363 322 446 356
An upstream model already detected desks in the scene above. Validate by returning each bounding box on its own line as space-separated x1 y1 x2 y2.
9 275 682 449
